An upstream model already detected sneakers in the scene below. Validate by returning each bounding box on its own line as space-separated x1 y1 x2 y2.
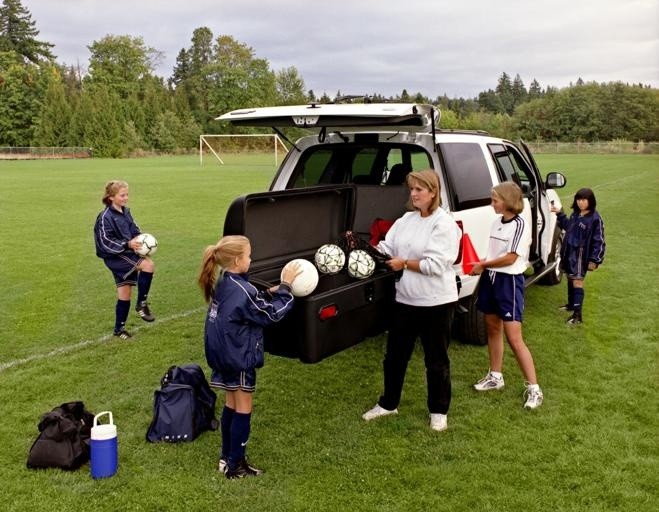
472 368 505 392
558 303 574 312
218 455 264 480
113 326 132 340
565 315 583 325
428 411 448 432
522 383 544 410
135 302 156 323
361 401 400 421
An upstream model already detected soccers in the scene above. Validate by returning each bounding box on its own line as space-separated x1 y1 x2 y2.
280 259 320 296
348 250 376 279
315 244 346 274
133 233 158 258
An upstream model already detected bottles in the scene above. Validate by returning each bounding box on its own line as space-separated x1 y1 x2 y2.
86 411 118 479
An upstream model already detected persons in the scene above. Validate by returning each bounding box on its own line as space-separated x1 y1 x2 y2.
361 170 462 432
196 235 304 479
467 181 544 410
94 180 156 341
550 187 606 324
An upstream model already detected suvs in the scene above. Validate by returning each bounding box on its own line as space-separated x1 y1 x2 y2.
213 98 575 350
